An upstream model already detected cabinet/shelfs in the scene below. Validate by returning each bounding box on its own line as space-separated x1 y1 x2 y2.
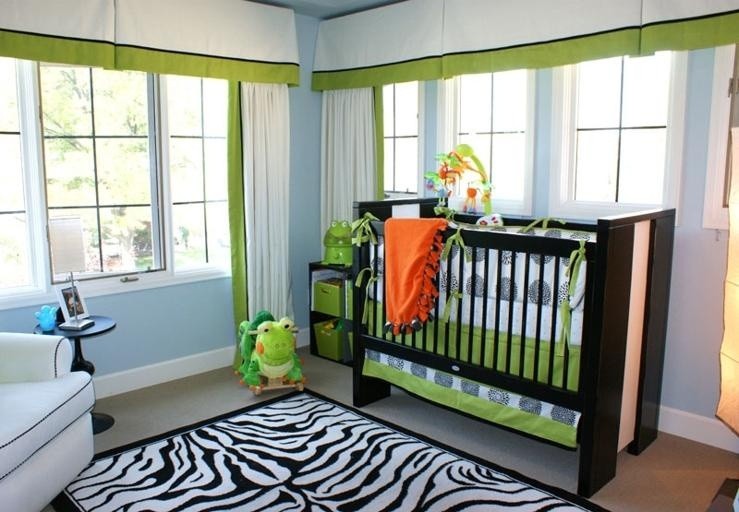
309 260 354 367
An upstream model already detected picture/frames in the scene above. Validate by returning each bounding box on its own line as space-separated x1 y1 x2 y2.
54 280 90 322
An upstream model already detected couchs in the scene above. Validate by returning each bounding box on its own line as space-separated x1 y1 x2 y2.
0 329 95 512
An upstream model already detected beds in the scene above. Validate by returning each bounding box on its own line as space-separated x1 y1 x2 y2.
351 196 676 498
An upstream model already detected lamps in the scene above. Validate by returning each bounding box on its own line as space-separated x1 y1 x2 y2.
714 125 739 438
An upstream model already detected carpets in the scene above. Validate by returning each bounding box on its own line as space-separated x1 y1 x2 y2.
50 386 614 512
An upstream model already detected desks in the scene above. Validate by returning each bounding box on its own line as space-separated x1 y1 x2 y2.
32 315 117 436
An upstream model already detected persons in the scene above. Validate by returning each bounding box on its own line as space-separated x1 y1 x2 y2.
66 293 79 308
